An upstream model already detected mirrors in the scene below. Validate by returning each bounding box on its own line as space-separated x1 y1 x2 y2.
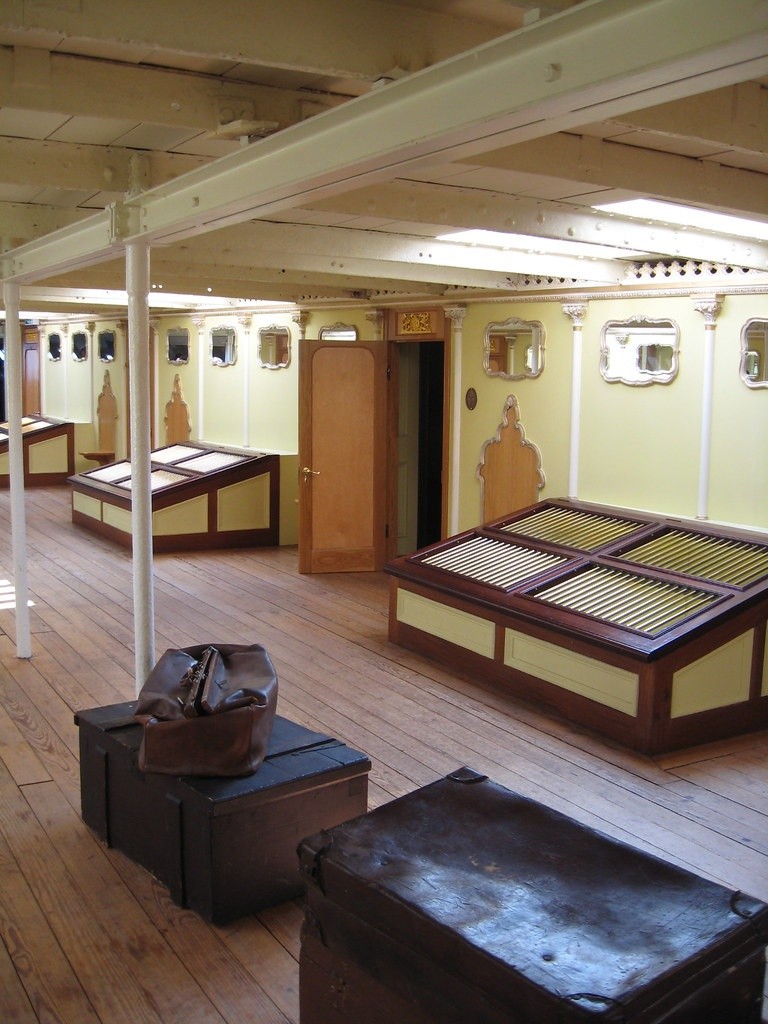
599 315 680 386
738 315 768 390
47 331 63 362
165 325 191 366
481 318 547 381
98 329 116 363
70 329 89 362
207 324 238 367
255 322 292 370
318 322 360 341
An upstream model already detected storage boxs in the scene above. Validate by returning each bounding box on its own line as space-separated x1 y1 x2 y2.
74 700 371 929
295 766 768 1024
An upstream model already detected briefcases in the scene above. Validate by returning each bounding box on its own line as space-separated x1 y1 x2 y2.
133 643 278 779
